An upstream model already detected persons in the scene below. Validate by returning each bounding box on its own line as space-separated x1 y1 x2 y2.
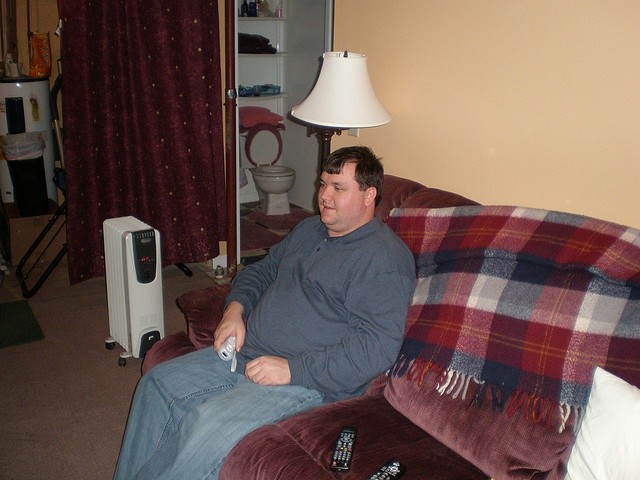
112 147 417 480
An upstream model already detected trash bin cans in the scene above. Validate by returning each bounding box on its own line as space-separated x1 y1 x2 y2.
4 131 48 215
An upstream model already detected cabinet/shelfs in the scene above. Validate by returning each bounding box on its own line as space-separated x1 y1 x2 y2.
239 0 286 129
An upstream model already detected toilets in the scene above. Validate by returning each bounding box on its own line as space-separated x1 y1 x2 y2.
244 122 296 216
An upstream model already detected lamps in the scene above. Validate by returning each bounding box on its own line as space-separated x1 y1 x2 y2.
289 50 392 165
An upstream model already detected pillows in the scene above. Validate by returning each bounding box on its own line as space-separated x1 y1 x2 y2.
564 366 639 479
383 359 582 479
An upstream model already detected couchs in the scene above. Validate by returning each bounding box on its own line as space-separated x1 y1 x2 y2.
140 175 640 480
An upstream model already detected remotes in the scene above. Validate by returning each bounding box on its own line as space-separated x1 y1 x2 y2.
331 427 357 472
368 458 408 480
